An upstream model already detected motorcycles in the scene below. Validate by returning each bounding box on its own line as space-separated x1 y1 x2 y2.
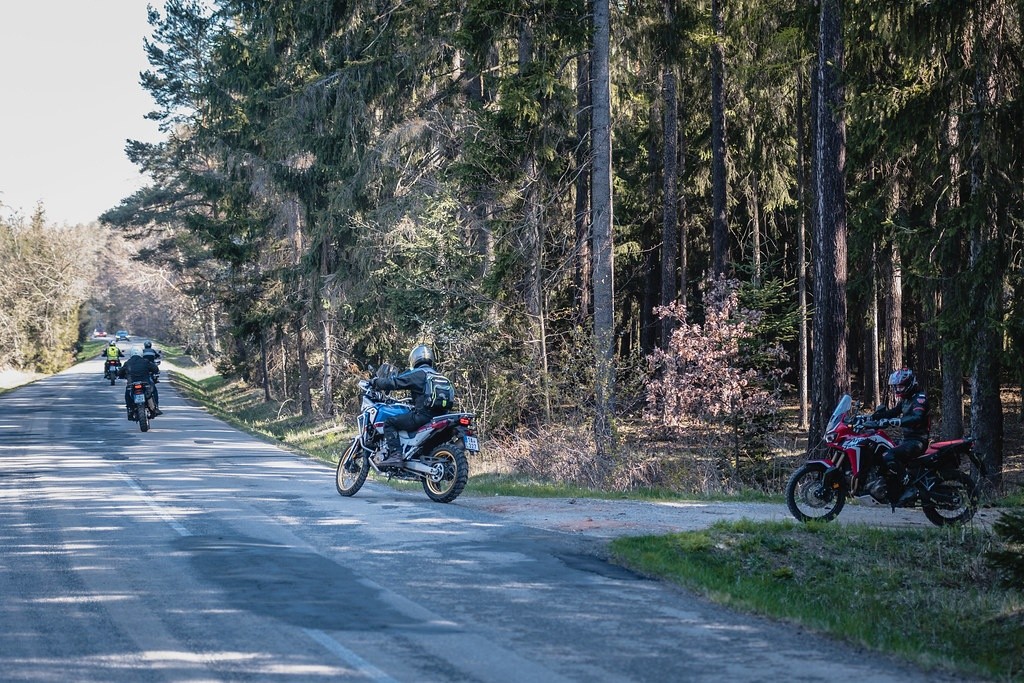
334 362 480 505
785 393 989 530
103 350 125 386
117 365 163 433
149 350 162 385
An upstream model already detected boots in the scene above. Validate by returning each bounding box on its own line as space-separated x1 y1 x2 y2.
378 451 404 468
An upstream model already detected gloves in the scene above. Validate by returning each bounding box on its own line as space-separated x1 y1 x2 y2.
853 416 870 425
368 376 378 388
874 418 901 428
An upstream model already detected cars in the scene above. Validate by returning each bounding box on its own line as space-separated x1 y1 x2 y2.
93 328 107 339
115 330 130 342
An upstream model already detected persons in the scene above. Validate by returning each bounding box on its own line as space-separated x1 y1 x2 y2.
860 367 930 498
368 346 455 469
101 339 125 378
117 345 158 421
140 341 161 374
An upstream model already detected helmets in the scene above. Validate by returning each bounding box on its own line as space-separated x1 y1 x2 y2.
130 346 143 358
887 367 921 399
109 339 116 347
144 341 151 349
408 344 436 371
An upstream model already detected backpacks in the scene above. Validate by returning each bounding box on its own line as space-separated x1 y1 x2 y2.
417 366 454 413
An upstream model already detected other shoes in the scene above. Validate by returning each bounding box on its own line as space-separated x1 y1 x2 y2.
891 485 918 507
